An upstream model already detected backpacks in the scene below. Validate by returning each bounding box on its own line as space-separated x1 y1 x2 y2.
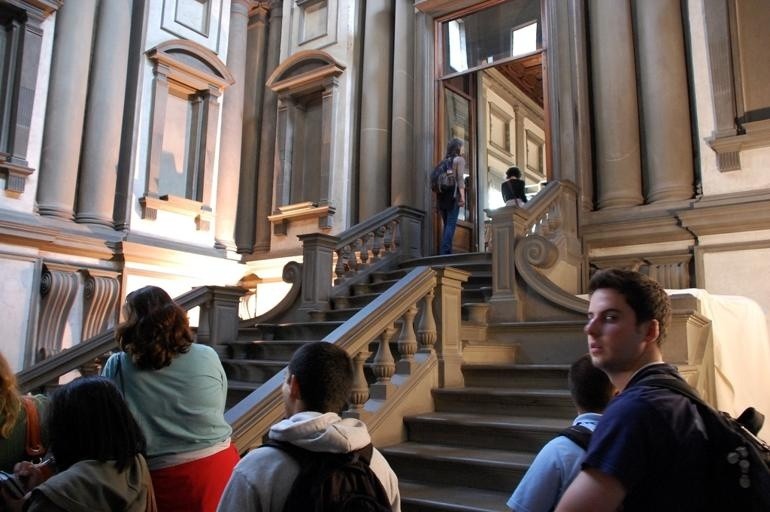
431 155 457 196
257 439 392 512
628 378 769 511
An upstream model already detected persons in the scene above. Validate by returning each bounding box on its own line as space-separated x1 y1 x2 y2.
0 351 157 511
431 138 466 254
551 270 730 511
508 354 611 512
216 341 403 511
100 286 241 511
501 167 526 209
